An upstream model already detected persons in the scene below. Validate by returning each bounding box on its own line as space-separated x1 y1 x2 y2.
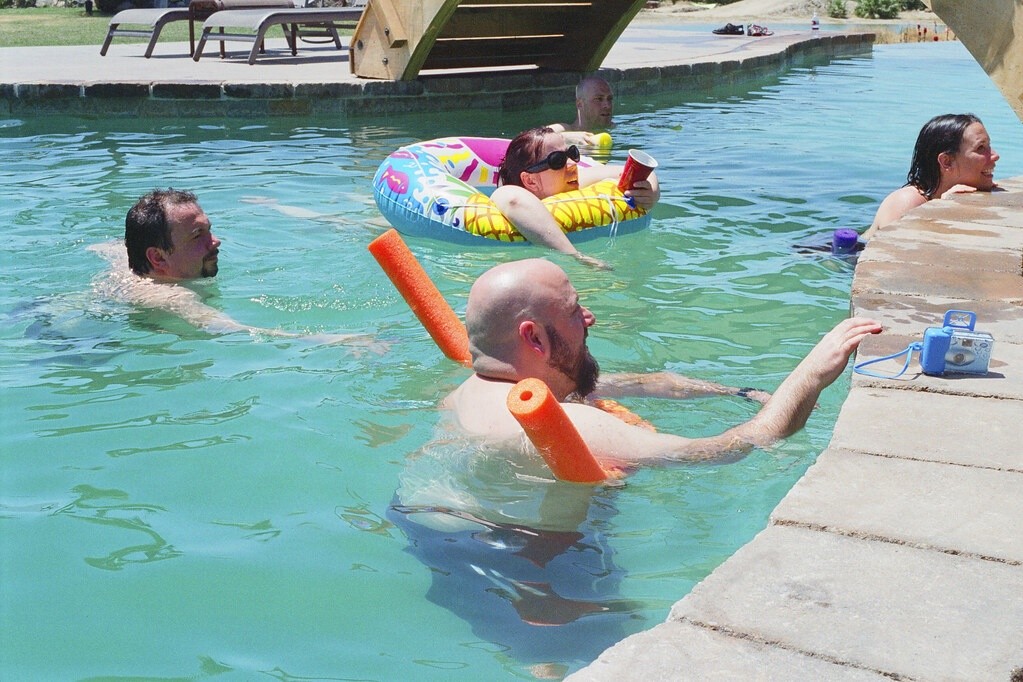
546 75 614 132
124 189 220 277
489 127 661 255
445 258 881 460
865 114 1000 235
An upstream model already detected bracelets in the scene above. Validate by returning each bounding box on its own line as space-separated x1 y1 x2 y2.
738 387 754 396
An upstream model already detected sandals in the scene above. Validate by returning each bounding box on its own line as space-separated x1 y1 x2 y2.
711 23 744 34
747 23 762 36
760 28 773 35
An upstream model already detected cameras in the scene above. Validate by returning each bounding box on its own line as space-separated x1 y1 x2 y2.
919 310 994 377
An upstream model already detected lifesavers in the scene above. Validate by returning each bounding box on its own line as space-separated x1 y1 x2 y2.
367 134 654 252
363 221 661 489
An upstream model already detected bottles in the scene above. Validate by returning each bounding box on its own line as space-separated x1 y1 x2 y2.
811 13 819 38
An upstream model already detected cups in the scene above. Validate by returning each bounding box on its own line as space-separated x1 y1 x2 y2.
619 147 659 195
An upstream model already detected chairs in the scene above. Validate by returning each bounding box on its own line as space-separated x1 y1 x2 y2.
100 8 190 58
193 0 367 65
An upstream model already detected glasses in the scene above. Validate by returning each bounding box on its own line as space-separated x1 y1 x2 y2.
527 146 581 175
